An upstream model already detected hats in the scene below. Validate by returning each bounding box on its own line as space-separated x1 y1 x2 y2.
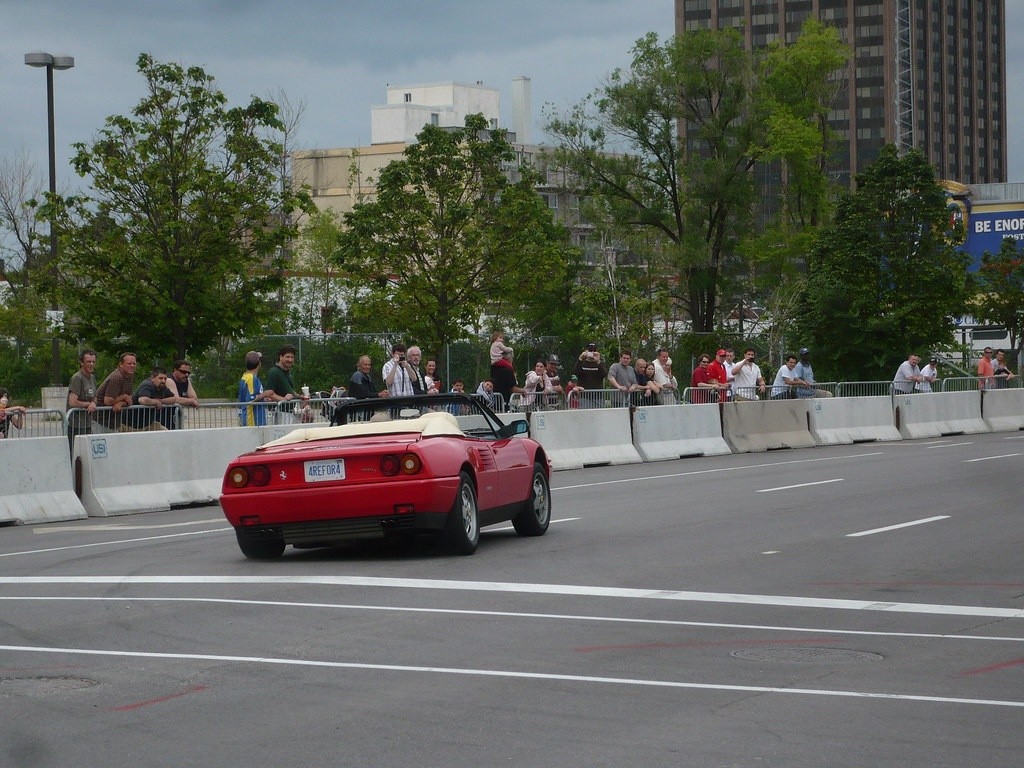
929 358 937 364
716 350 727 356
799 348 810 355
587 342 598 350
546 354 560 364
245 351 263 364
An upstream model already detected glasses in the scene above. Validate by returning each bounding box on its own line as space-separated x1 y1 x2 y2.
588 349 597 352
176 368 191 375
550 363 557 365
986 352 993 354
702 360 709 364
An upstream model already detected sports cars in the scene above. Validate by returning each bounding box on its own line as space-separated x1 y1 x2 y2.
219 393 552 561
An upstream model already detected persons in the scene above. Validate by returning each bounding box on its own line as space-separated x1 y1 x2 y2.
91 352 137 433
471 379 496 410
166 360 199 429
489 331 563 413
382 343 444 419
64 351 97 468
572 342 607 409
446 377 470 415
607 347 679 408
266 347 314 425
564 374 585 409
889 355 940 408
770 348 815 400
132 367 177 432
238 350 274 427
0 386 27 440
976 347 1015 416
347 355 390 422
691 347 766 404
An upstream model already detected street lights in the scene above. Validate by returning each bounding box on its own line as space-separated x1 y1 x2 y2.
24 52 75 387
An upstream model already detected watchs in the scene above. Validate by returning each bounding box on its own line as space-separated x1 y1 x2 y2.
760 384 765 387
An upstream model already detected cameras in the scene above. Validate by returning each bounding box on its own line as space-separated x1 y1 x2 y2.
399 356 406 362
586 352 594 357
749 357 756 362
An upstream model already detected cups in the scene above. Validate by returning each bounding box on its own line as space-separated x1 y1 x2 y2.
301 387 309 399
